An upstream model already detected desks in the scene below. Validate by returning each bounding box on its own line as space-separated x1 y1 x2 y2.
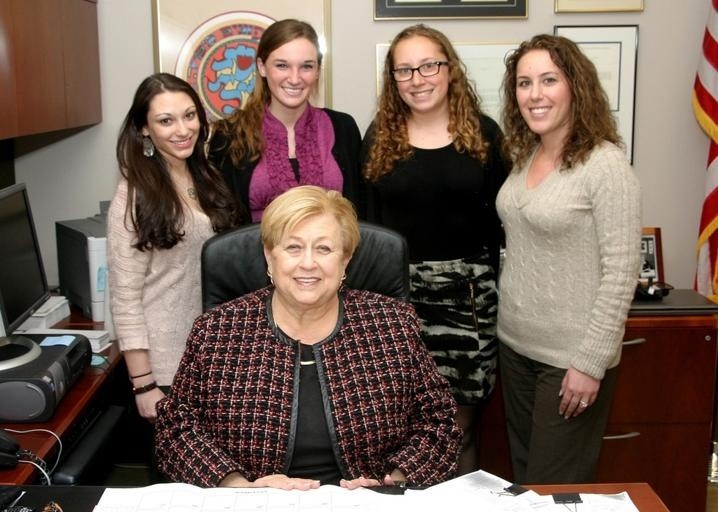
514 483 668 512
0 316 123 483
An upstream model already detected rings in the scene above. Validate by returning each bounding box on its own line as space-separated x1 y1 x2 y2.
579 400 588 408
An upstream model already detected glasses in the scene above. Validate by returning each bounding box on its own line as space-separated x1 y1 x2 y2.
392 61 449 82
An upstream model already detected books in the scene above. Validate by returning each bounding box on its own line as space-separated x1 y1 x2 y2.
93 467 640 511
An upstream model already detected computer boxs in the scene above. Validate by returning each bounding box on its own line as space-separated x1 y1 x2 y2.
0 334 92 423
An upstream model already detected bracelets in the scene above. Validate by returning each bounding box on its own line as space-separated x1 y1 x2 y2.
128 371 152 382
132 380 156 396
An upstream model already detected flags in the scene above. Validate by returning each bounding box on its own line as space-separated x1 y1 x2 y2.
687 1 718 308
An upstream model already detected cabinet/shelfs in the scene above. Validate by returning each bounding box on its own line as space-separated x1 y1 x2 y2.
600 288 718 512
0 0 103 139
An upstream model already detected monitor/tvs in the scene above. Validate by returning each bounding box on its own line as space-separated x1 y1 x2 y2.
0 183 51 337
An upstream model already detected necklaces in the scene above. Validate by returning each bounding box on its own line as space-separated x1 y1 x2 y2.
170 169 198 200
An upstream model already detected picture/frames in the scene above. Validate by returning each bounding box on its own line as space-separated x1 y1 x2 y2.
375 42 523 133
150 0 331 124
553 23 639 166
554 0 644 13
371 0 529 20
638 226 663 289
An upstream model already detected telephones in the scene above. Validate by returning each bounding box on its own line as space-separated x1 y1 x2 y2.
0 429 20 469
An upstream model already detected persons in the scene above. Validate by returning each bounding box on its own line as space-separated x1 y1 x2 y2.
105 72 252 466
150 183 466 492
202 18 366 230
357 21 510 482
494 32 644 484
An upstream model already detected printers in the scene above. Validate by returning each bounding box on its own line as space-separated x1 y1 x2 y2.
55 200 112 322
